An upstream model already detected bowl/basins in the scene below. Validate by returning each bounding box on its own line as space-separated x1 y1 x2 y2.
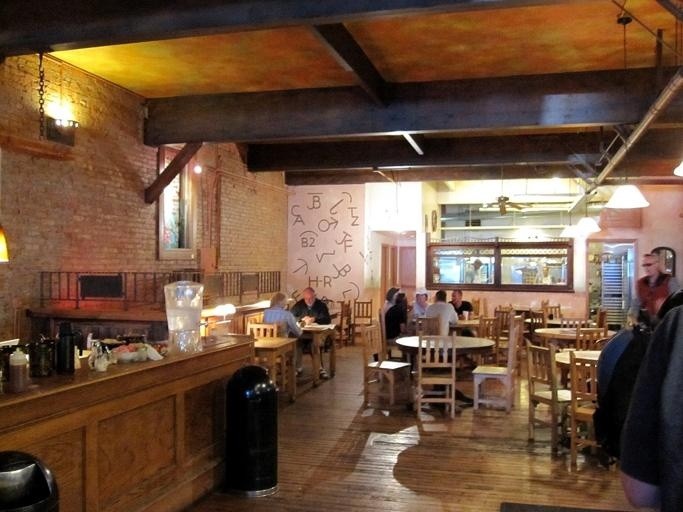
115 351 139 366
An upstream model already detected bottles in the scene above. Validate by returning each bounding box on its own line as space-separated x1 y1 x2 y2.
54 317 77 376
8 347 28 394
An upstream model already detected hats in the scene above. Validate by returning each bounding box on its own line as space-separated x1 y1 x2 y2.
386 287 399 300
414 288 431 301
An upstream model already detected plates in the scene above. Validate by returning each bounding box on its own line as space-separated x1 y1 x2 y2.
293 320 331 329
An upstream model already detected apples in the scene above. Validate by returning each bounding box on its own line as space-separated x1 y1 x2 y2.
117 342 146 353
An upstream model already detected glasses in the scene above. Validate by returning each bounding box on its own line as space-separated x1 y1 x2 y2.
642 262 655 267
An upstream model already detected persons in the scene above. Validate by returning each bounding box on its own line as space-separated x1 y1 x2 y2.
263 291 311 380
447 288 477 337
410 288 429 336
424 289 458 337
384 292 416 380
592 252 682 511
533 265 553 284
288 287 330 379
381 287 413 337
464 258 482 283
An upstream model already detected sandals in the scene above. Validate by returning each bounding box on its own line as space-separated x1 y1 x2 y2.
319 368 332 380
295 369 304 377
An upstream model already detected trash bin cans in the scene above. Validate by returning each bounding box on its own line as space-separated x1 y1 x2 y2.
227 366 278 496
0 450 58 512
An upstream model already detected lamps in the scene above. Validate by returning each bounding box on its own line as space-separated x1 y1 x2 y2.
574 185 601 233
602 9 651 211
44 106 79 147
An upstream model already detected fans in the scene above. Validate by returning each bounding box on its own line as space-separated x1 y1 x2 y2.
478 166 526 216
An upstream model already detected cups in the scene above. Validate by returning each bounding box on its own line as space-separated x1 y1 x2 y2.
462 311 469 321
29 338 58 376
431 237 568 257
